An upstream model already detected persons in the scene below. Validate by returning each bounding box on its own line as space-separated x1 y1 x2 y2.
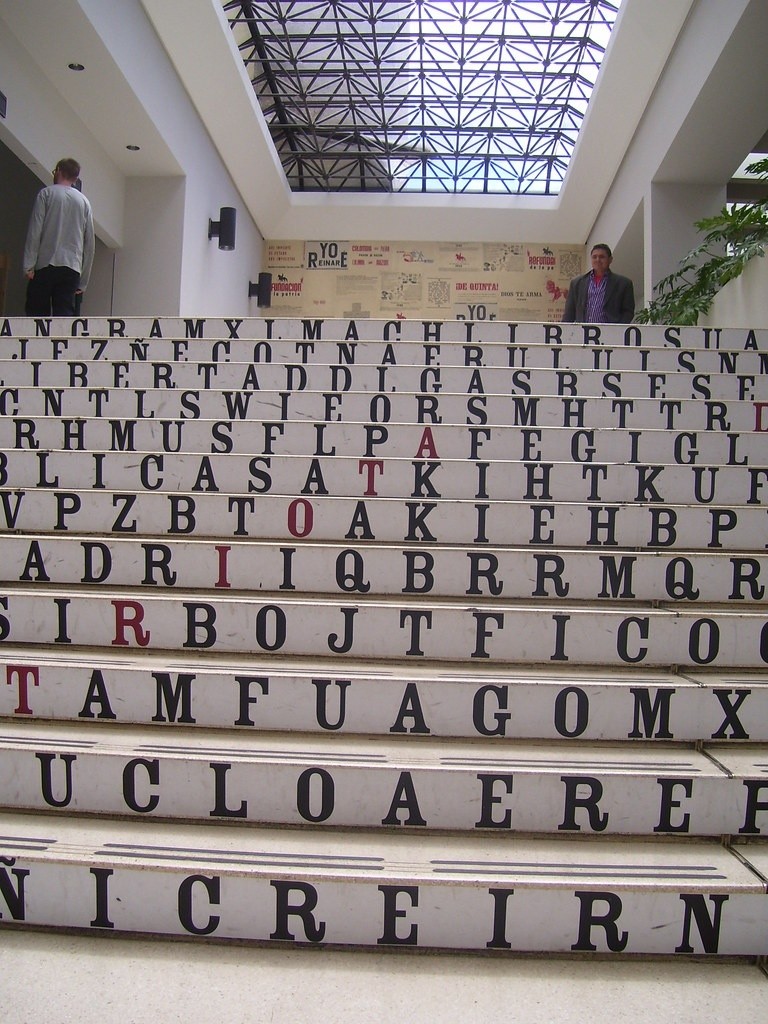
24 158 95 316
561 244 635 323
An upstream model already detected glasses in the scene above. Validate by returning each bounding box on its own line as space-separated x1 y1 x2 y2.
52 168 56 175
